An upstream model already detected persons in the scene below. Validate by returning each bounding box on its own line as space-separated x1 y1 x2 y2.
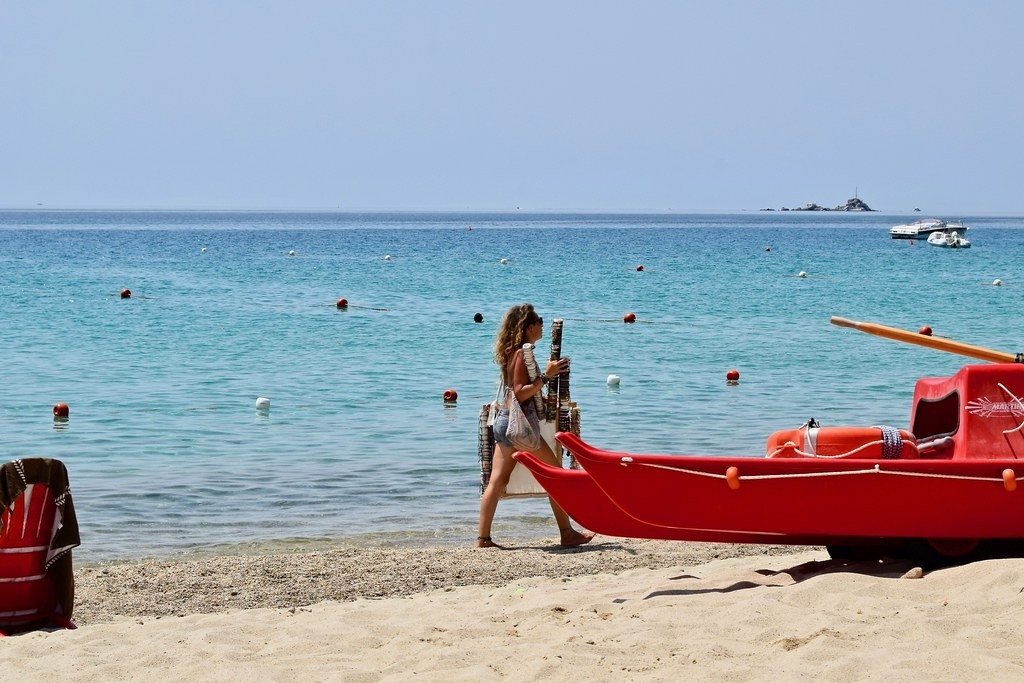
475 302 597 550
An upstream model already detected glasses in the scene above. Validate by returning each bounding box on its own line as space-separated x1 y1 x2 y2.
536 317 543 324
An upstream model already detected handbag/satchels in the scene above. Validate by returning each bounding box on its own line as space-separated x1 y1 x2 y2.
487 380 503 426
505 390 540 452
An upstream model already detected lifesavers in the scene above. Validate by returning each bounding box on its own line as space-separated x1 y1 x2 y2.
766 425 918 460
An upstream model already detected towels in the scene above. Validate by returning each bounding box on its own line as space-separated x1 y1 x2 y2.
0 456 81 636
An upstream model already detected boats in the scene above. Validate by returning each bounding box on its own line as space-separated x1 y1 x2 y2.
926 231 971 248
890 217 970 240
512 410 827 543
555 315 1024 545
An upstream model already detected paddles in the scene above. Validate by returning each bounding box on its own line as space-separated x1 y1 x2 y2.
830 315 1024 364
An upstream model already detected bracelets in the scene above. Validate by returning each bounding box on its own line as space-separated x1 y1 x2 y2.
539 372 550 384
